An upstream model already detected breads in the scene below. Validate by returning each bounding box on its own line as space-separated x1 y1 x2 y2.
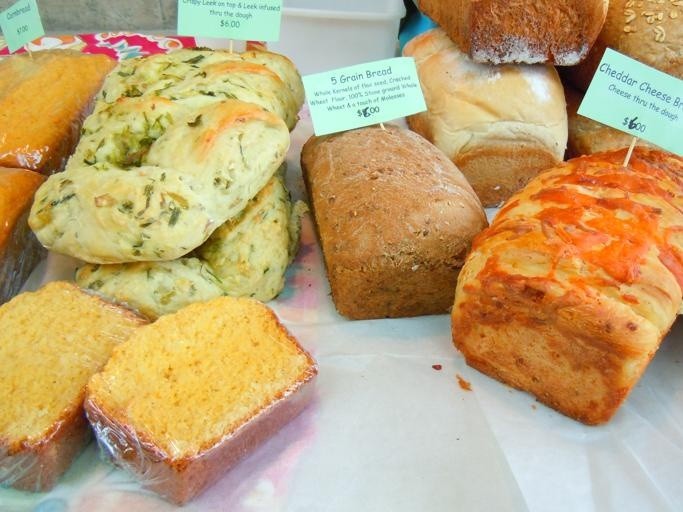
0 49 121 308
0 280 319 507
300 1 682 424
27 48 305 319
